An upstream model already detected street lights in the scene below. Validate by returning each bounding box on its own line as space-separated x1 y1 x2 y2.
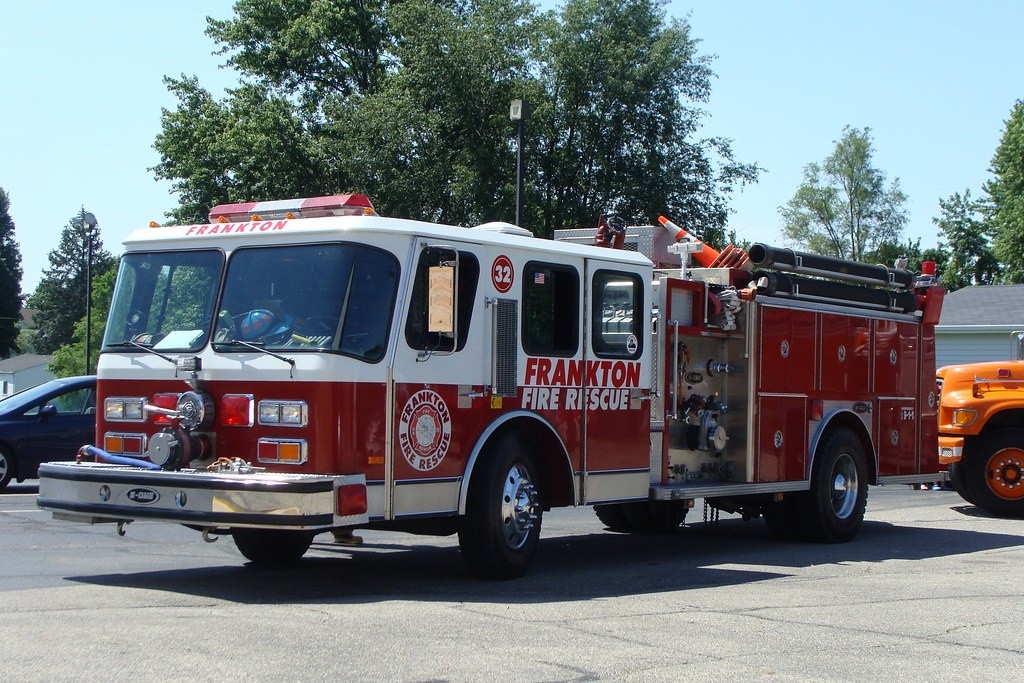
509 98 525 225
83 212 98 375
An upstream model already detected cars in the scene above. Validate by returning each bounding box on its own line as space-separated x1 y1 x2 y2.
0 375 97 492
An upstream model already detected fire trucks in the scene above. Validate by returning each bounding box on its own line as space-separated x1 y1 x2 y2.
36 190 951 580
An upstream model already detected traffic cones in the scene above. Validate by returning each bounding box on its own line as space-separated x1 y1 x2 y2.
657 215 750 269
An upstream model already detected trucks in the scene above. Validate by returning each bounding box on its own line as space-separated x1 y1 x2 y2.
937 360 1024 518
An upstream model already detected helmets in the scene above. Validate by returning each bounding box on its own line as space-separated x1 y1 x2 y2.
240 308 294 342
